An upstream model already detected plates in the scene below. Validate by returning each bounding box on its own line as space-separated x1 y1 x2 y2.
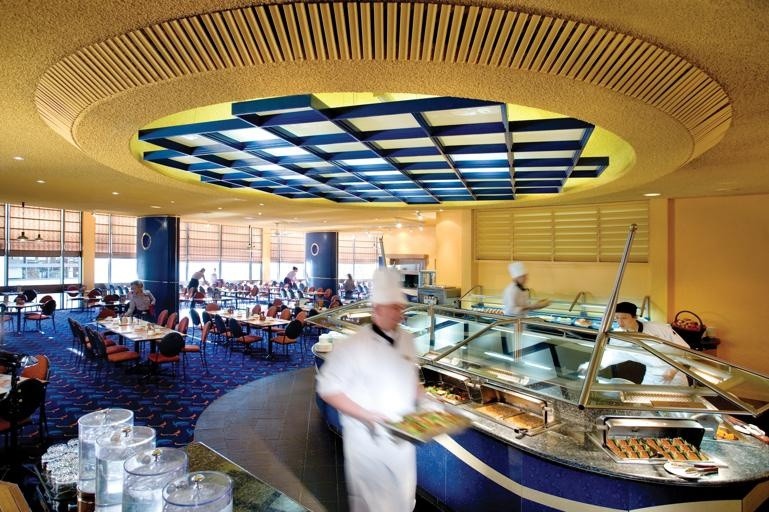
663 461 708 480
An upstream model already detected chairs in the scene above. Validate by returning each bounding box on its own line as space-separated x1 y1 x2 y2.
190 309 216 342
25 296 54 330
181 321 211 368
239 305 261 335
340 280 372 296
124 286 129 295
207 286 212 294
202 311 217 345
154 312 177 352
194 292 204 304
104 294 119 313
244 286 250 295
0 303 14 334
229 283 233 289
177 317 189 369
206 303 225 341
263 308 290 350
117 286 123 294
148 304 154 315
147 332 184 389
303 286 308 293
181 288 187 293
273 298 282 307
246 279 305 289
280 288 286 297
233 285 237 291
315 287 324 297
271 319 303 359
141 310 168 354
288 289 294 299
93 330 140 388
237 283 242 290
214 313 231 357
216 281 221 288
249 306 277 337
199 285 204 294
251 286 258 296
309 286 314 293
23 288 36 302
321 289 332 306
297 291 302 299
268 303 272 309
24 300 59 333
278 311 307 352
280 305 287 311
0 349 15 374
88 290 101 316
225 282 228 288
295 307 303 319
85 325 128 383
308 309 321 336
73 319 116 373
110 285 114 294
21 354 49 379
212 289 221 298
68 317 98 360
229 318 262 367
96 309 117 332
1 377 48 455
69 285 80 309
188 287 194 297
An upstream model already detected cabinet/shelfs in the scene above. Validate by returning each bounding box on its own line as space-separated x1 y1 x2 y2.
403 270 461 308
306 296 769 512
460 287 651 334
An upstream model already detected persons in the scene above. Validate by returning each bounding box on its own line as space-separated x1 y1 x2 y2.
315 270 443 512
188 268 209 291
284 266 298 288
121 280 156 322
576 302 691 387
343 273 354 294
503 263 539 316
